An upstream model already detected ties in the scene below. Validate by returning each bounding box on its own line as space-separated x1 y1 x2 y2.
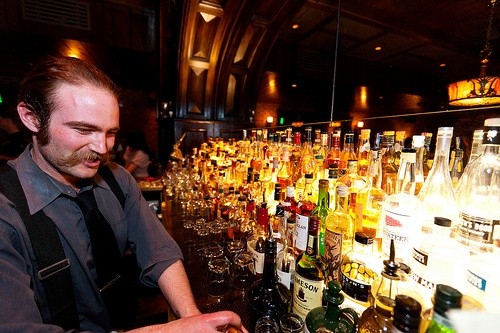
72 189 125 320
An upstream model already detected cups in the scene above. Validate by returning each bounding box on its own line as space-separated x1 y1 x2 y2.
208 228 223 246
204 221 215 229
217 222 229 243
255 315 279 333
226 240 246 260
232 251 255 298
280 313 305 333
161 166 189 228
194 229 209 256
208 259 231 296
215 216 226 224
192 224 202 232
195 217 206 224
204 245 224 264
183 220 194 241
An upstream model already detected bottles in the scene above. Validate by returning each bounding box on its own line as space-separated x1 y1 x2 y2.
189 118 500 333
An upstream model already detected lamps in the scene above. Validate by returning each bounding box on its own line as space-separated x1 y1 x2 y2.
447 0 500 107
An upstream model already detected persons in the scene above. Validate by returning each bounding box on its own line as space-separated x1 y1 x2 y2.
0 56 249 333
124 129 149 179
0 115 32 157
